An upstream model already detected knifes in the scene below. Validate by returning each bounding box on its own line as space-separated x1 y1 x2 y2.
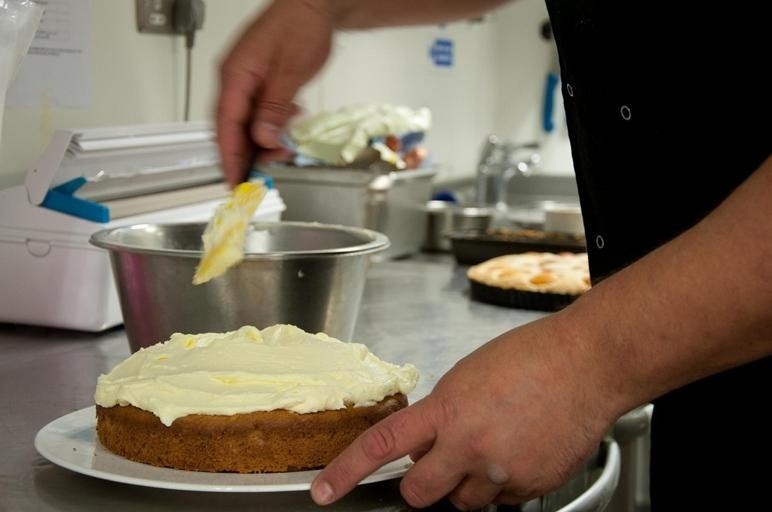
190 107 307 293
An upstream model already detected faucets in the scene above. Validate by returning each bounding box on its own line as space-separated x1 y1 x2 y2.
473 138 527 217
498 137 541 215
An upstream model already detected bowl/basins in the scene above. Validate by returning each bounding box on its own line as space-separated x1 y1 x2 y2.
444 227 587 268
89 220 390 363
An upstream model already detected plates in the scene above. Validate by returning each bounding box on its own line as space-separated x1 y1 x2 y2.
33 378 416 495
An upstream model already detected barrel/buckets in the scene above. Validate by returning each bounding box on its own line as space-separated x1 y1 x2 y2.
546 200 584 235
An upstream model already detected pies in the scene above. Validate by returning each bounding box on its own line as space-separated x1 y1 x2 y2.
464 249 592 296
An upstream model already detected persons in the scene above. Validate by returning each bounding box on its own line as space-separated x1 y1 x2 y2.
215 1 772 508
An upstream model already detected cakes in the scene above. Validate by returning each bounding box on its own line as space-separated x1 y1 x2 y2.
94 325 419 475
